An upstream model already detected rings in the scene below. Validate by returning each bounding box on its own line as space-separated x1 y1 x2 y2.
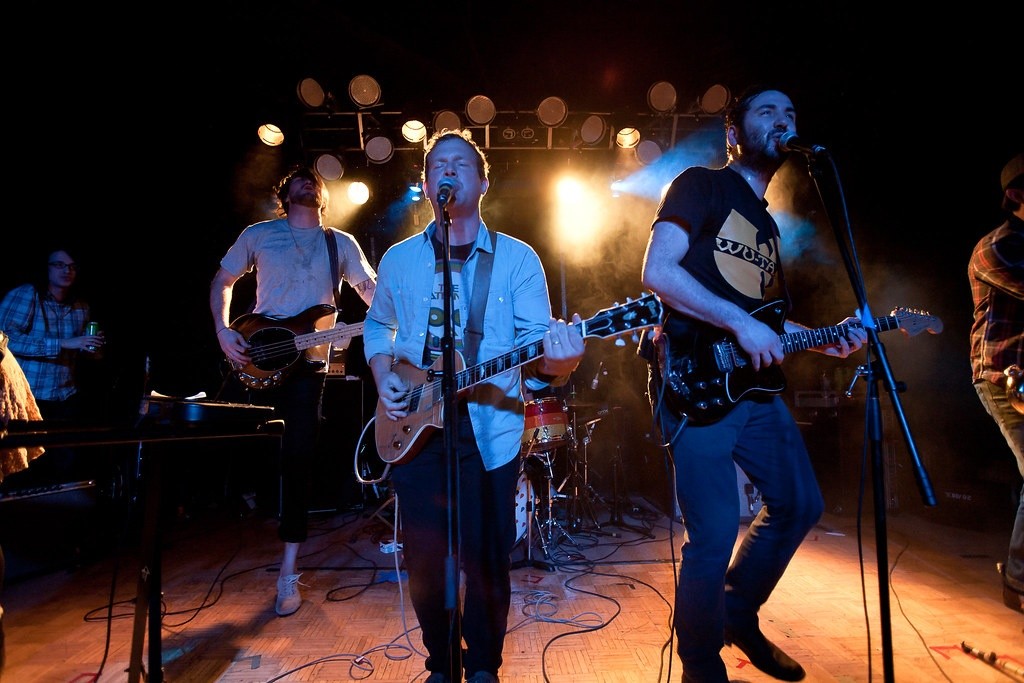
552 342 561 346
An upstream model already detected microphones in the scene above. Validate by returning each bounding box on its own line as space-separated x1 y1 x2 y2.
779 132 831 157
437 177 455 203
591 363 602 389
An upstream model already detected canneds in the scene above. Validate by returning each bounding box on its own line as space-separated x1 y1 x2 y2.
85 322 99 352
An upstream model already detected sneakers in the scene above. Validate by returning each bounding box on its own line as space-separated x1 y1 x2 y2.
275 571 311 616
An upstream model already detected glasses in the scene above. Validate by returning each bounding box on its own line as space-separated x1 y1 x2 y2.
48 261 77 271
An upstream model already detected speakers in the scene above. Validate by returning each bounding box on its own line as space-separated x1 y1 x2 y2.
277 378 365 512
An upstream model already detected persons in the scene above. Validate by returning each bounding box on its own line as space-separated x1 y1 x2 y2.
362 129 588 683
0 251 105 420
211 169 378 615
966 155 1024 593
636 89 870 683
0 330 47 486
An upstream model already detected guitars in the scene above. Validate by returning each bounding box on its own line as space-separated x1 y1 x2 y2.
227 302 367 389
656 297 945 425
374 290 663 464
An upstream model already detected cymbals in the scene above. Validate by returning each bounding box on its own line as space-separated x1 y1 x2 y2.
564 398 596 408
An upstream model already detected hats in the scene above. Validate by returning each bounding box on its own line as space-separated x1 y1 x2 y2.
1001 156 1024 210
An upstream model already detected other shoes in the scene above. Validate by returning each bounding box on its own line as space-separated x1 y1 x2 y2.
724 612 806 682
423 670 499 683
995 563 1024 614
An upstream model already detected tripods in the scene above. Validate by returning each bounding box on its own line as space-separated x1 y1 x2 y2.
511 366 657 572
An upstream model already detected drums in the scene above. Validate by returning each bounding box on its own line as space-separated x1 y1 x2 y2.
510 470 538 548
520 395 574 454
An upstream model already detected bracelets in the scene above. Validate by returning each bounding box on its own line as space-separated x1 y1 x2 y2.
216 327 227 336
